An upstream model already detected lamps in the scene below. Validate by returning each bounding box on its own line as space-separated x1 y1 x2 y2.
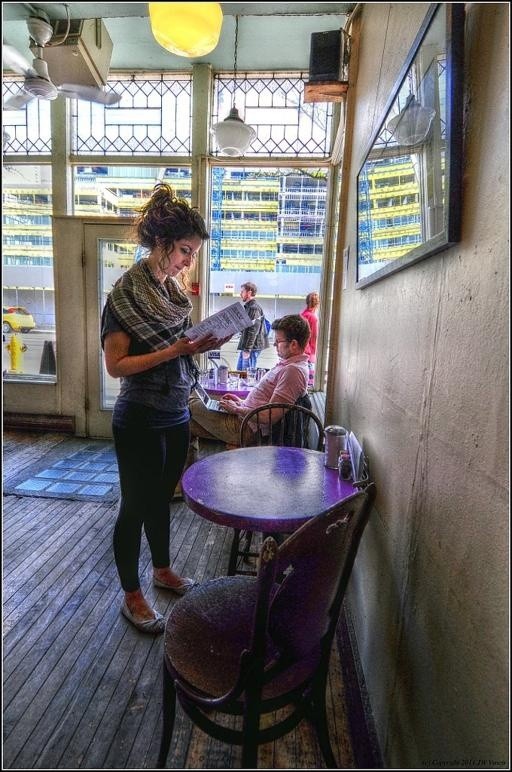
386 66 437 146
145 1 224 58
209 14 259 159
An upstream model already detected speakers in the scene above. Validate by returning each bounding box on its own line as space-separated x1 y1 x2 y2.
309 29 345 82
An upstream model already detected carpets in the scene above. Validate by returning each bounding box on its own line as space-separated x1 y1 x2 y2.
4 439 122 503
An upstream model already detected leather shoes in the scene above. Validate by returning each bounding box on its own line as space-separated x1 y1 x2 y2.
118 596 168 634
152 575 201 596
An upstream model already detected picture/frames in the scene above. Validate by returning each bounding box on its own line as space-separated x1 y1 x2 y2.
351 2 459 292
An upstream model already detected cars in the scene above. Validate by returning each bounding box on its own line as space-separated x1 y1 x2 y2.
3 305 35 334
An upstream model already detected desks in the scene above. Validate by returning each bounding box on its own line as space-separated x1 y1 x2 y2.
197 376 256 401
179 445 365 545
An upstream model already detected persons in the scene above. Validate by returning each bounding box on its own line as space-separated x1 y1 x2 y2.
96 179 233 635
300 293 319 386
172 315 312 497
236 283 269 371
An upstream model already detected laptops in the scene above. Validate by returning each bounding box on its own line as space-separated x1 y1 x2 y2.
193 381 239 416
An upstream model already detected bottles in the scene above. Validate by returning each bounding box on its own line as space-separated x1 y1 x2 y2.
338 450 351 480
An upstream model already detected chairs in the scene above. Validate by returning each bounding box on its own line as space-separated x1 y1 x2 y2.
221 402 326 579
151 477 378 772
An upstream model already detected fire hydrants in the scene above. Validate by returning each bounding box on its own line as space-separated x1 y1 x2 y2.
5 334 28 372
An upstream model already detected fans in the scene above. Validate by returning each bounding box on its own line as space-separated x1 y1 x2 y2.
3 2 121 112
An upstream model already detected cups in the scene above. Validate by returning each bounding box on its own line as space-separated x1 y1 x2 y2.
322 425 348 469
191 282 200 295
199 364 267 387
